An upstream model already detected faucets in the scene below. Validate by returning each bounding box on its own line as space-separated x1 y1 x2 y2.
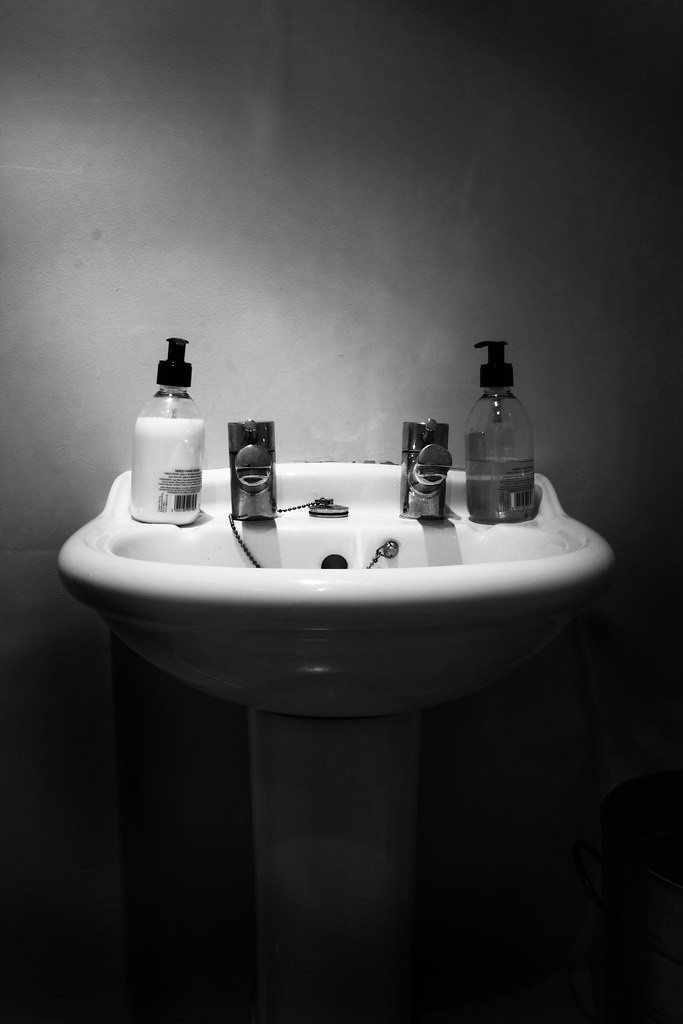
400 419 453 519
227 419 278 522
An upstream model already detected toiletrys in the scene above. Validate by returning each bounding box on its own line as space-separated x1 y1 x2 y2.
464 340 536 524
130 338 205 526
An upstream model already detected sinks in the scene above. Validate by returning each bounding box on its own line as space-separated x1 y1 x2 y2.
56 522 612 717
